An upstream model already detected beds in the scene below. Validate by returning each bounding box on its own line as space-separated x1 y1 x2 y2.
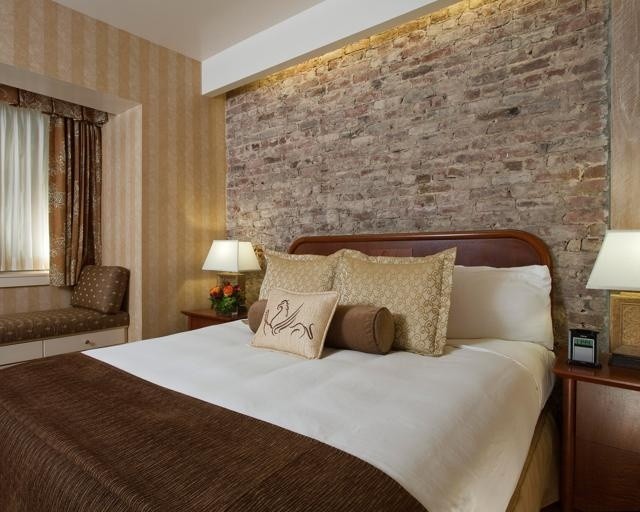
0 230 560 512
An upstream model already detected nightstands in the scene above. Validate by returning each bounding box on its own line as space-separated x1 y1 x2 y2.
181 308 248 330
552 347 640 512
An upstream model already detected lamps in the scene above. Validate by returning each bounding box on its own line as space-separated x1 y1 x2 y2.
586 230 640 368
202 240 262 312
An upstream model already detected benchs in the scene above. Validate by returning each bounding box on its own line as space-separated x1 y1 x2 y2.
0 306 130 370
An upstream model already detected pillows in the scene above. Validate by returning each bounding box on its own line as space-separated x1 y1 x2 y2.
69 265 128 315
247 245 457 361
